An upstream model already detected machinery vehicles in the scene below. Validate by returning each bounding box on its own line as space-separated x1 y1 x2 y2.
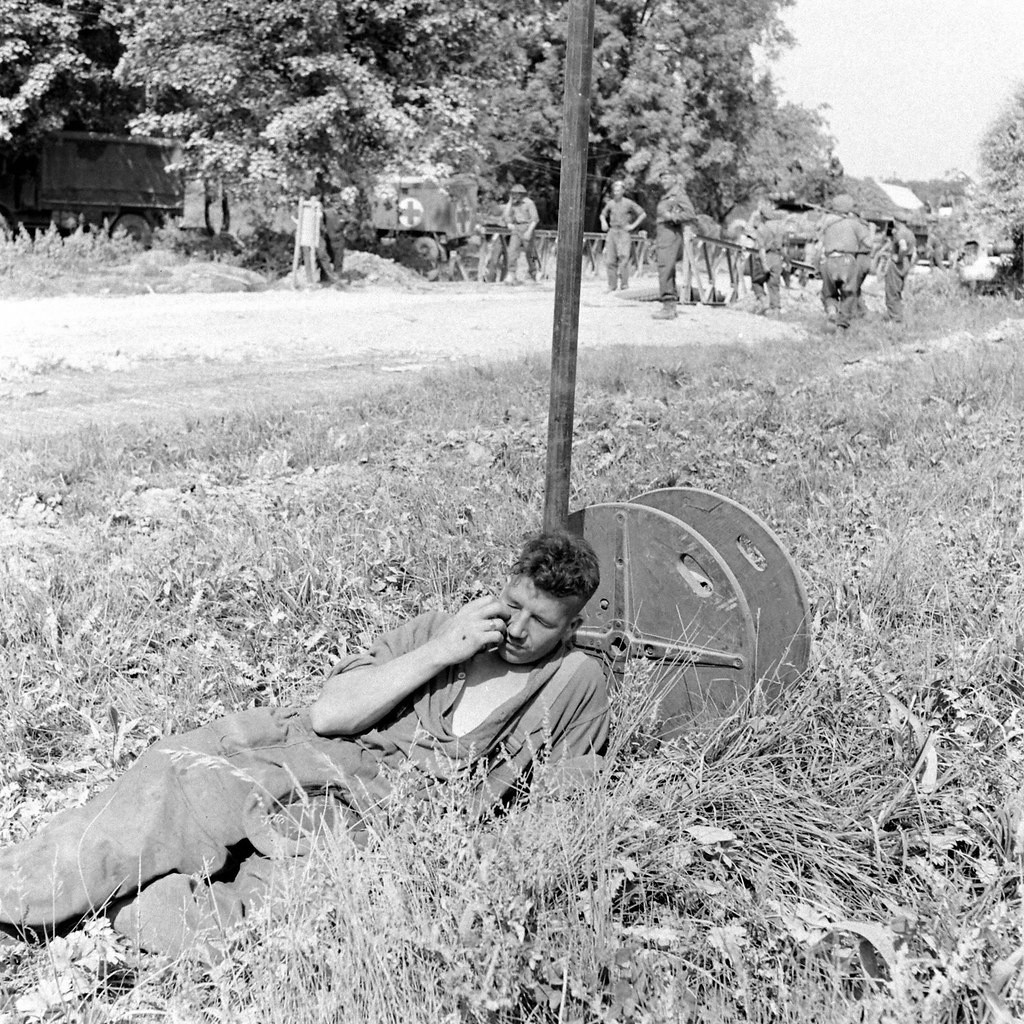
0 129 185 254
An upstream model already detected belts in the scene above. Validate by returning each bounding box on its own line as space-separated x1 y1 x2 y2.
514 221 529 225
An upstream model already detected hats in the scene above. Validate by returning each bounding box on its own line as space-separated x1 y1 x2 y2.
611 180 627 188
894 211 907 223
658 169 678 178
510 184 528 193
760 206 775 219
831 193 854 213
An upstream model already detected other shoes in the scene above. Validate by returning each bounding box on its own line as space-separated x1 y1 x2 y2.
503 275 519 287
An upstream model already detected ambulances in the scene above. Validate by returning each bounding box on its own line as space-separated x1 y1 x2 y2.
335 172 479 271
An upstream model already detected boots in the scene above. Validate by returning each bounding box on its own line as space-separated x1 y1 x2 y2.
652 298 680 320
755 294 771 315
824 305 838 336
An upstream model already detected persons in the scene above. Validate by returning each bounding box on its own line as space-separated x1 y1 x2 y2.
499 185 539 286
293 168 912 330
600 182 644 292
650 171 697 319
1 532 610 958
